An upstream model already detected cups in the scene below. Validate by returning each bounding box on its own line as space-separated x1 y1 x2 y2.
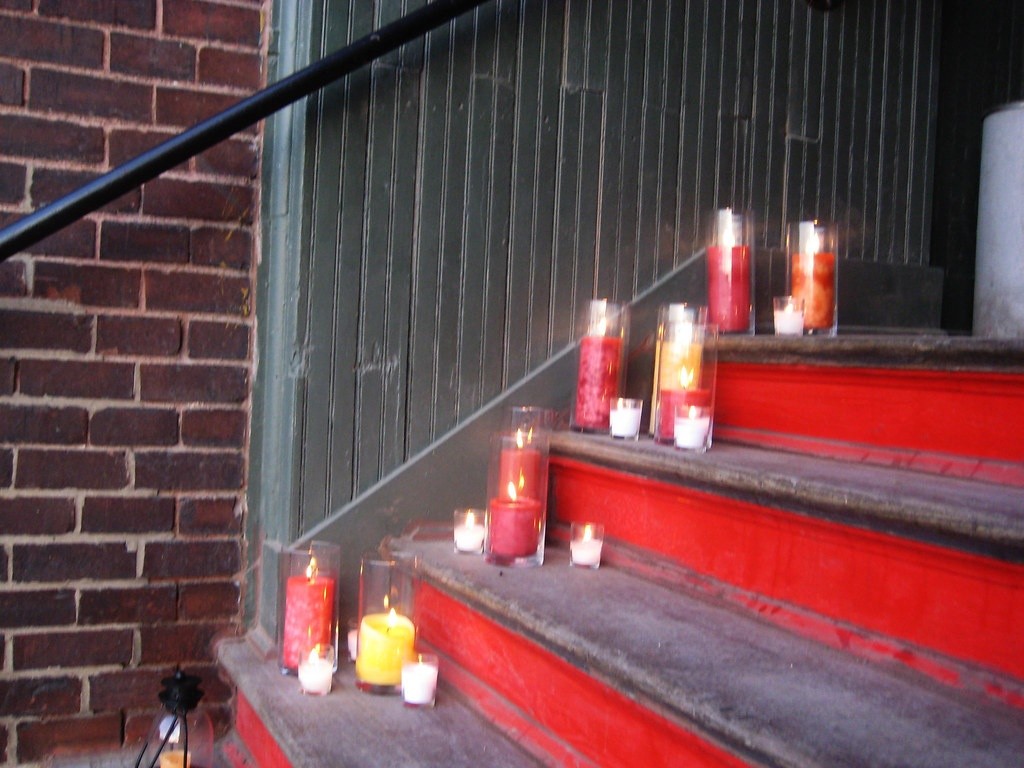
346 616 357 664
569 519 605 570
609 397 644 442
652 323 719 449
275 540 341 676
569 298 632 437
671 404 715 455
354 542 423 695
772 296 806 338
783 218 839 338
647 301 709 440
483 406 552 568
400 654 439 710
298 643 335 697
454 507 486 557
704 207 757 339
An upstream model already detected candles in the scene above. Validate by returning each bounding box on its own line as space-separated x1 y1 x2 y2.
609 395 643 443
769 219 840 338
279 538 438 709
567 296 632 433
452 405 606 567
647 208 754 453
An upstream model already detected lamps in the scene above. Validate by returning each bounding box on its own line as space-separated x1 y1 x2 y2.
134 661 214 768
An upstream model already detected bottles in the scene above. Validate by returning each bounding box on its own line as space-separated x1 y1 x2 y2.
147 665 213 768
